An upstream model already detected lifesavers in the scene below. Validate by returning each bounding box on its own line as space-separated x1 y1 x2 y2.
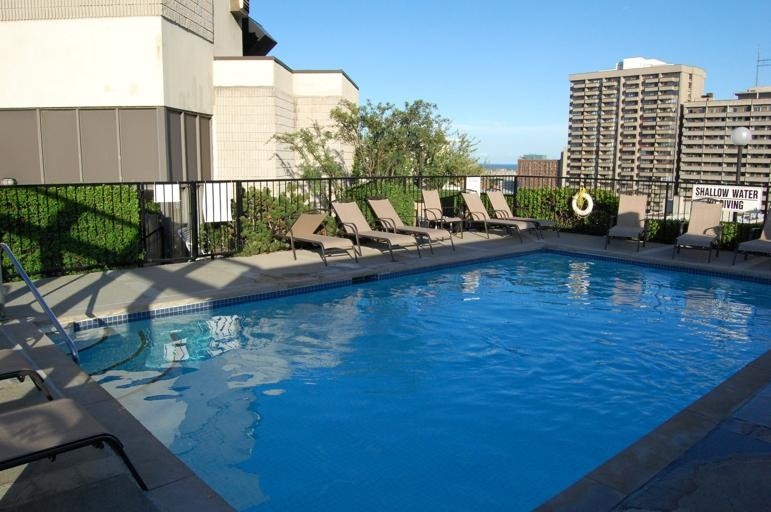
572 192 593 218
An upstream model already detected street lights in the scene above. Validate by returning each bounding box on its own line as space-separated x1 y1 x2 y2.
731 127 752 222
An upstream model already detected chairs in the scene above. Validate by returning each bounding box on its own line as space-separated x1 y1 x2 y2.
732 207 770 266
604 194 648 253
0 342 150 489
672 197 723 264
279 188 544 267
